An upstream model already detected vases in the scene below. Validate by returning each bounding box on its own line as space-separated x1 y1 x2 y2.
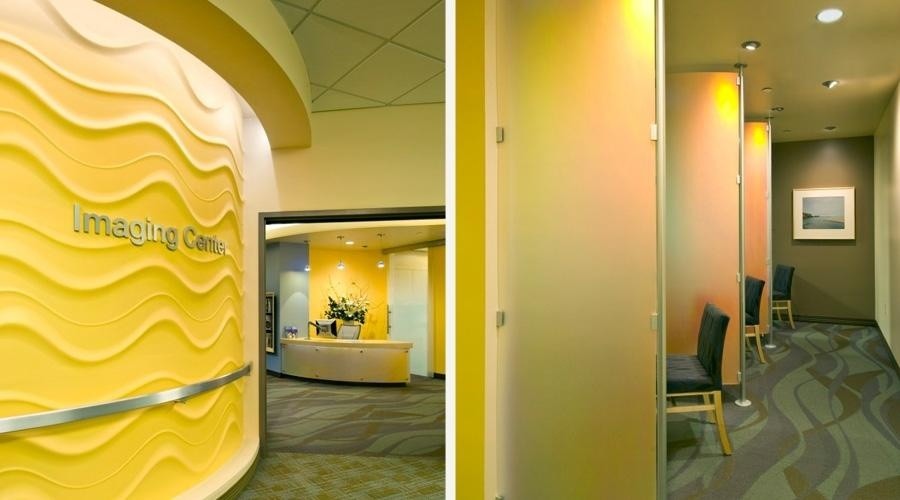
343 320 354 326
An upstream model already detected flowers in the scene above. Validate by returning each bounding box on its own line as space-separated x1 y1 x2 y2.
324 282 380 324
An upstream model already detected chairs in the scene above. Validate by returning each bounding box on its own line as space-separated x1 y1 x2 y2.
666 264 796 455
337 324 361 340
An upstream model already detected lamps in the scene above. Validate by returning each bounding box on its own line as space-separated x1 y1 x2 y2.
304 233 384 271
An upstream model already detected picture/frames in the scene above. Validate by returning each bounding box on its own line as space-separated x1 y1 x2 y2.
792 186 856 241
266 291 275 355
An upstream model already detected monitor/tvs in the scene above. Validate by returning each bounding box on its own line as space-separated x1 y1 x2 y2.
316 320 337 336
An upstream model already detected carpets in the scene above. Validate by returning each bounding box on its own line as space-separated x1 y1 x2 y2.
265 377 445 459
